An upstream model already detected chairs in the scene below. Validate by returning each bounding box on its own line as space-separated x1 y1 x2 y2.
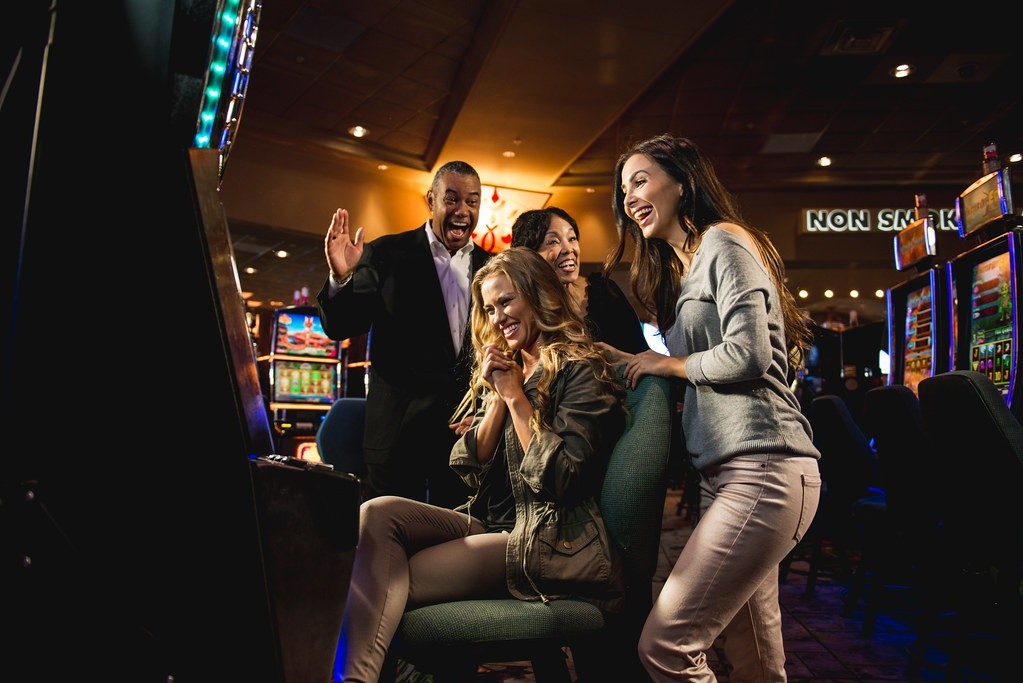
315 370 1023 683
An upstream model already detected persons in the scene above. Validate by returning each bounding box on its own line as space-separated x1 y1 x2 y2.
323 162 499 505
332 246 625 683
510 208 650 356
605 133 822 683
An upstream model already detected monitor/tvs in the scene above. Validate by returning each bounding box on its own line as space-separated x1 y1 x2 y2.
901 283 932 400
968 250 1011 401
273 359 337 405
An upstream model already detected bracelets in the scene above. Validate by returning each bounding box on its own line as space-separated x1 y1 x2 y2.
334 276 351 284
